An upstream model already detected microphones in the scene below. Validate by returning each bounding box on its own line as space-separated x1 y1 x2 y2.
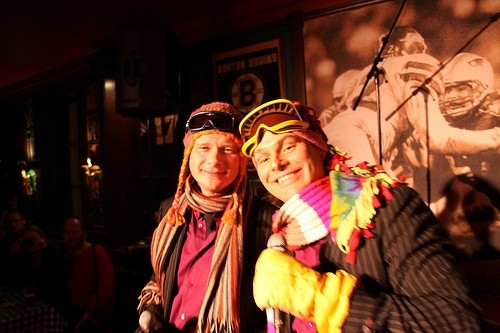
267 233 288 333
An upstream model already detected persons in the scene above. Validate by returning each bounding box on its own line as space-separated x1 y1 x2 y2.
239 96 487 333
59 216 116 333
0 209 49 286
317 26 500 175
134 100 280 333
383 52 500 251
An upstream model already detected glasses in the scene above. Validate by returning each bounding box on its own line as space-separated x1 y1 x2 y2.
185 111 239 133
242 114 310 158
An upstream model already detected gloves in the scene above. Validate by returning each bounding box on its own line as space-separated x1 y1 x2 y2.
252 247 358 333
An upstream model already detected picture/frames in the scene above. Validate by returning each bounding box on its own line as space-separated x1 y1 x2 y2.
291 1 500 211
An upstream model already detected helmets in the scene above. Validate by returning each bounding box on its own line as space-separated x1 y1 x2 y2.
376 27 427 56
333 69 361 99
441 53 494 116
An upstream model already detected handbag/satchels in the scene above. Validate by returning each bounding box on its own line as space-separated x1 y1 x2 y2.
94 307 122 329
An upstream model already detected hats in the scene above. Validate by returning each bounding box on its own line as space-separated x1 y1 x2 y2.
167 102 247 226
239 98 332 162
